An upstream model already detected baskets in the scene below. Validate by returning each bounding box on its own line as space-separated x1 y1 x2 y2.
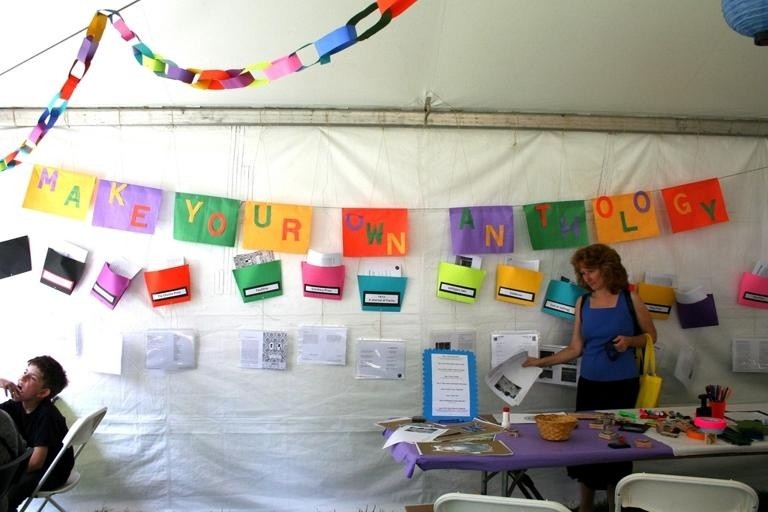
535 414 578 441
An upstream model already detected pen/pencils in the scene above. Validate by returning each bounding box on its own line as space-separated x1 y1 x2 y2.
706 384 732 402
438 432 460 437
618 408 674 419
414 441 423 456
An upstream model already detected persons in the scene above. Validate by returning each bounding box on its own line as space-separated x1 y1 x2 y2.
522 244 657 512
1 356 75 512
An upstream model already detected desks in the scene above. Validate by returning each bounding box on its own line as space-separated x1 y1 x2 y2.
382 402 768 497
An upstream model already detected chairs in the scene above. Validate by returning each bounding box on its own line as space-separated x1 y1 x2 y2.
0 447 33 512
614 470 759 512
19 406 109 512
433 489 572 512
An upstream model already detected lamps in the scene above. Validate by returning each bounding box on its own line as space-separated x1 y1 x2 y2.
721 0 768 46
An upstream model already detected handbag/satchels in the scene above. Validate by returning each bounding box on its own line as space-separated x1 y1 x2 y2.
635 334 664 408
